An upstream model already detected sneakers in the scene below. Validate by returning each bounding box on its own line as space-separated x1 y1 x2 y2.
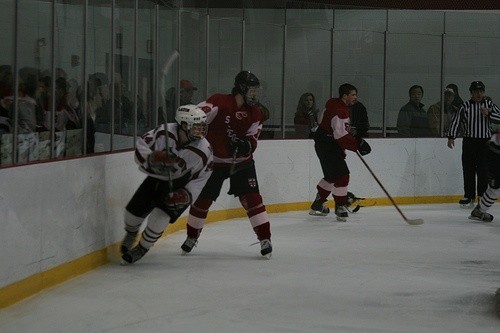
180 237 198 255
121 241 150 265
335 206 348 223
250 239 272 259
469 206 494 222
459 197 475 209
309 205 330 216
119 229 138 255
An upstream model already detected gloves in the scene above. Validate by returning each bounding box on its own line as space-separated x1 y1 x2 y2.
230 139 253 157
358 139 371 157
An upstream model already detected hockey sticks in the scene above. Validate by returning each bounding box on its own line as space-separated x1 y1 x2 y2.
355 148 425 225
159 49 181 197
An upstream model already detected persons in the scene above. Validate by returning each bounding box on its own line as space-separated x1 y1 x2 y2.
180 70 273 259
0 65 198 163
348 101 370 137
120 103 213 266
294 92 320 140
467 134 500 221
428 83 464 135
397 85 428 136
447 81 500 208
309 82 372 222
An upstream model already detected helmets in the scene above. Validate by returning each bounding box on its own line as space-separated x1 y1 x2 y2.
176 104 207 131
235 71 259 95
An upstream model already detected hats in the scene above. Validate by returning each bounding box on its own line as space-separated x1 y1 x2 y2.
469 81 485 91
181 80 198 92
